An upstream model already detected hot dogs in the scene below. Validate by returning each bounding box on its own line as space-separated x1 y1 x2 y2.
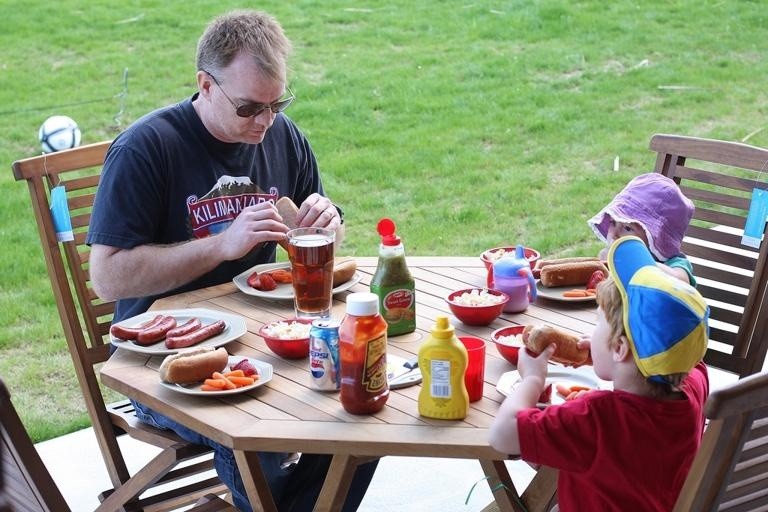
523 323 593 368
532 257 609 288
274 197 299 252
160 346 228 384
332 256 356 289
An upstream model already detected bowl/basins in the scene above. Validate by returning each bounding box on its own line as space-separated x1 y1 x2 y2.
481 247 541 274
257 318 315 361
443 287 509 327
491 327 549 366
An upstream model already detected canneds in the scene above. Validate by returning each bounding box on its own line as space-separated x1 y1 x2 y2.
309 317 341 392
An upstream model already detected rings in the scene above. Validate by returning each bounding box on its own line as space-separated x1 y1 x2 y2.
324 209 333 217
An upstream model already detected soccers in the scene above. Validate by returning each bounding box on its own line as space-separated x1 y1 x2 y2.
39 116 81 153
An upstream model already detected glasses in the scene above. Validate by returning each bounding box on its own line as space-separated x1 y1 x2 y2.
197 67 296 118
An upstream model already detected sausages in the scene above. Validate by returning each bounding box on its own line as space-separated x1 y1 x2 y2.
111 315 225 348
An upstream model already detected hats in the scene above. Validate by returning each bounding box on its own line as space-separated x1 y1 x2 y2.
605 234 712 385
586 171 696 262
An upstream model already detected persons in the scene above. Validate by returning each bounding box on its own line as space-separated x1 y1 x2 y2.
585 172 696 289
487 234 710 512
84 7 379 512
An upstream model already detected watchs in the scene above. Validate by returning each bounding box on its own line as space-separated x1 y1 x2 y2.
332 203 344 225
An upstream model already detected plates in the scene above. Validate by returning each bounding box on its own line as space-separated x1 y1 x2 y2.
160 359 277 398
531 279 597 303
383 353 423 391
109 307 248 356
233 262 362 300
495 368 610 410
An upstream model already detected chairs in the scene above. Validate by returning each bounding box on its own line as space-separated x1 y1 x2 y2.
1 376 230 510
639 132 768 376
677 377 768 511
12 128 289 510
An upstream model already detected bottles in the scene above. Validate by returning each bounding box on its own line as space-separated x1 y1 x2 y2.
491 246 538 315
337 293 390 418
415 317 470 422
370 220 417 342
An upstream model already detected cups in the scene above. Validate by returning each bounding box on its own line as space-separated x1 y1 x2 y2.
286 226 335 323
455 336 485 403
